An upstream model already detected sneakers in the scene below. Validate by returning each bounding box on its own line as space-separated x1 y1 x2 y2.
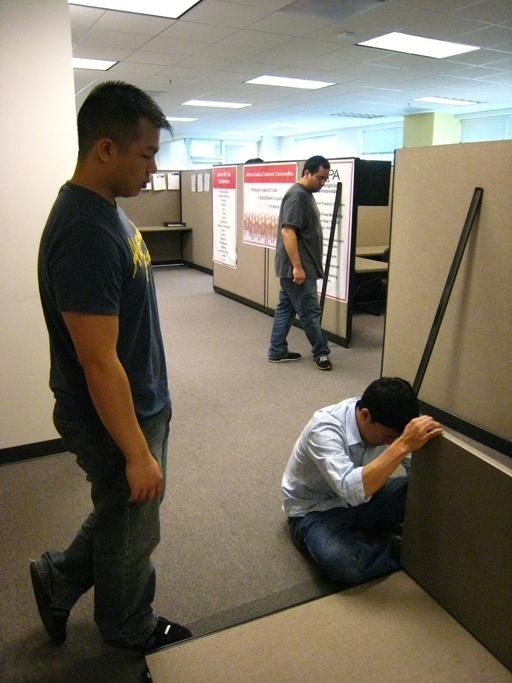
102 615 192 655
28 557 71 646
268 350 302 365
312 352 332 372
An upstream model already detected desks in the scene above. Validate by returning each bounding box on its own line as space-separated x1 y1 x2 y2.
139 226 192 267
355 245 389 256
355 256 388 317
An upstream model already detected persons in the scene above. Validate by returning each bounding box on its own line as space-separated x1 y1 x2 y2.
281 377 443 587
27 80 192 654
267 155 333 371
